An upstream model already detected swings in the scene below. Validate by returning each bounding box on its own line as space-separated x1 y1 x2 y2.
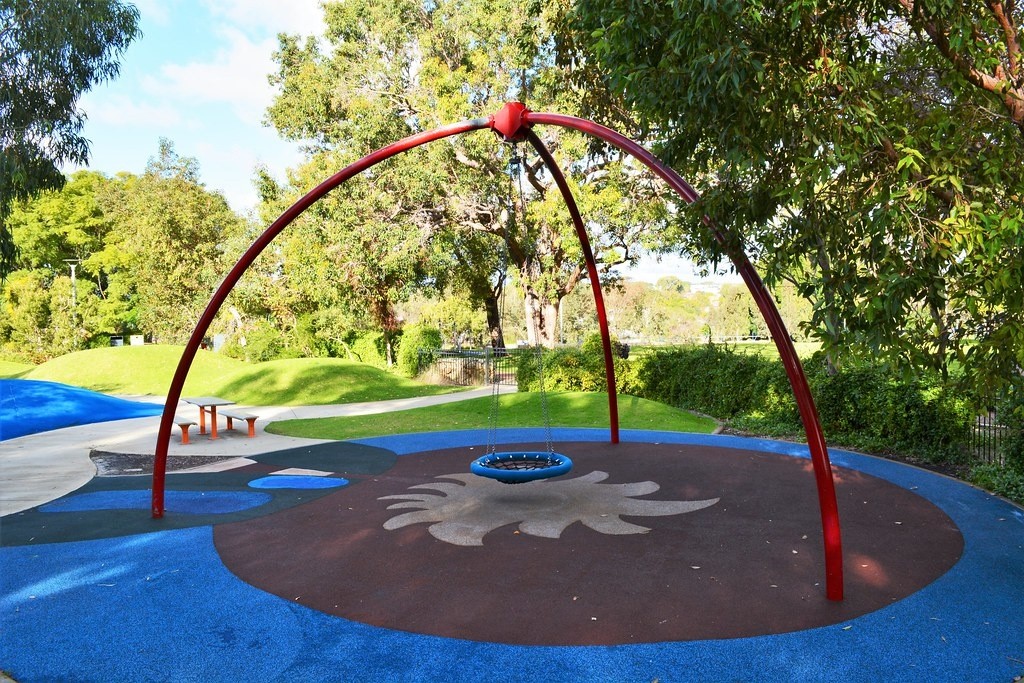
470 142 573 484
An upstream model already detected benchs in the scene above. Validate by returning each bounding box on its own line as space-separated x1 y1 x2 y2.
160 416 197 443
219 411 259 437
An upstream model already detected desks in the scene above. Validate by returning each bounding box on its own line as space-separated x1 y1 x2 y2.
182 397 236 439
